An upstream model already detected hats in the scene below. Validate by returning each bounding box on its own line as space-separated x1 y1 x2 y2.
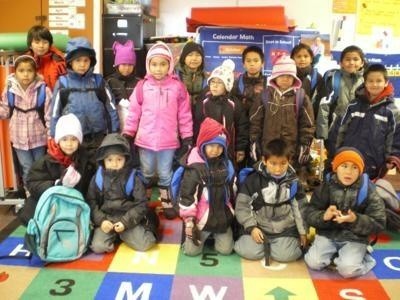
64 37 97 69
206 59 235 91
54 115 83 144
268 57 302 92
181 42 206 67
199 132 231 161
331 147 366 174
146 41 174 77
112 41 137 67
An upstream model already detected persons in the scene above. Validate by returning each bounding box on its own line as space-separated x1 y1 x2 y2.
173 42 209 100
236 140 307 262
170 119 236 256
335 63 400 178
249 57 314 164
0 57 55 197
107 40 142 99
51 38 119 171
305 145 387 278
232 46 270 110
316 46 370 176
121 42 192 218
290 44 321 99
24 27 66 94
193 59 249 167
19 114 93 229
91 134 161 254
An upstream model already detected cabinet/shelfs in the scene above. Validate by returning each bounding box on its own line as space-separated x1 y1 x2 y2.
101 13 157 80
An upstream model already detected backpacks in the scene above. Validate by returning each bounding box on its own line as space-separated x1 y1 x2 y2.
26 184 91 263
370 157 400 235
